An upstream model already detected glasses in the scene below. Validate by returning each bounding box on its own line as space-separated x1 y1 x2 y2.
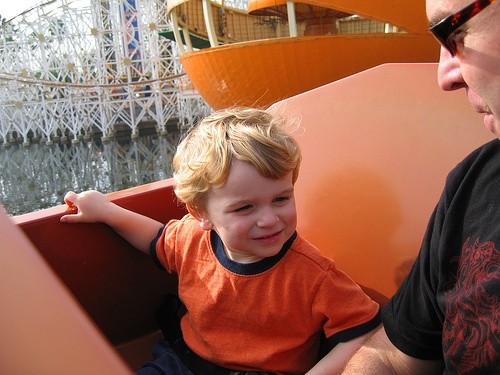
428 0 497 59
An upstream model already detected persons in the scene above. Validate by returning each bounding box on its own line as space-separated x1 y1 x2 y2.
343 0 500 375
58 105 381 375
